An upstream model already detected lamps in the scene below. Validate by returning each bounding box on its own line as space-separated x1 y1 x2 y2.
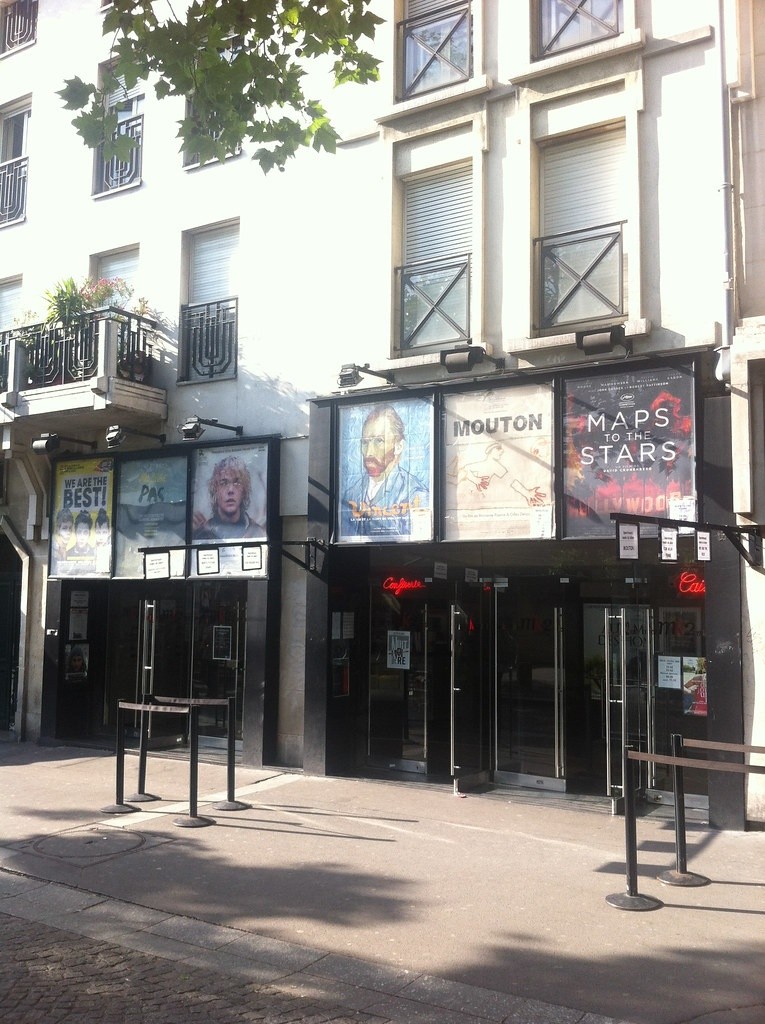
104 425 165 449
574 326 633 356
339 363 395 388
30 433 97 454
179 416 242 441
440 347 504 372
